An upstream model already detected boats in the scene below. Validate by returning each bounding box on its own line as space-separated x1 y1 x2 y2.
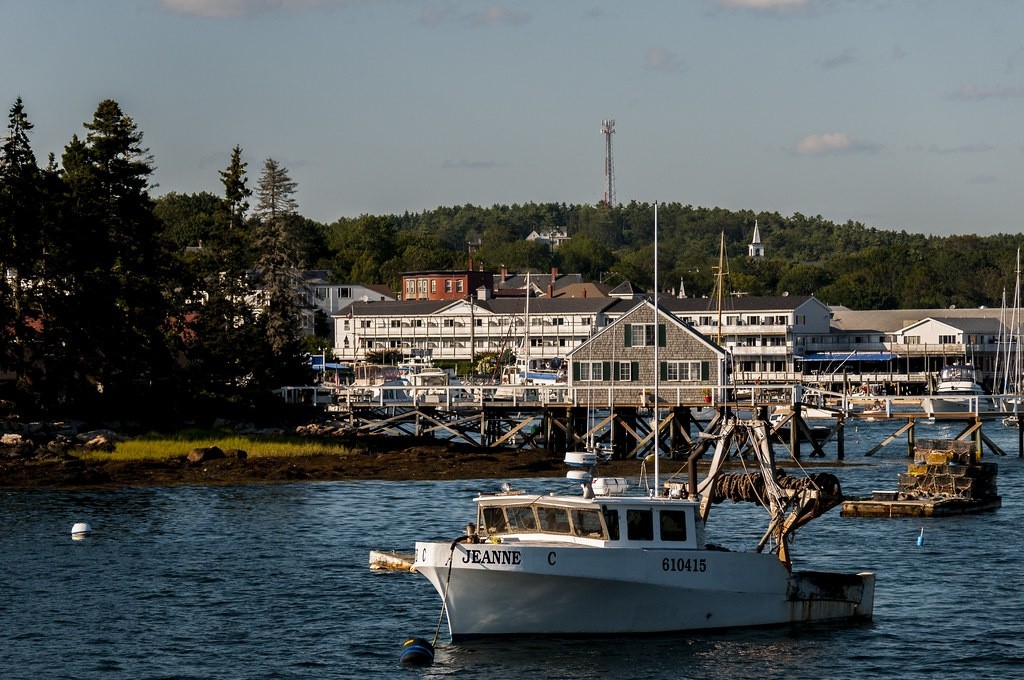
919 354 992 420
765 402 833 442
367 205 881 646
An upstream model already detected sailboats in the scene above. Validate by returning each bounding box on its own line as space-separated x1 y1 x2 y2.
991 246 1024 414
